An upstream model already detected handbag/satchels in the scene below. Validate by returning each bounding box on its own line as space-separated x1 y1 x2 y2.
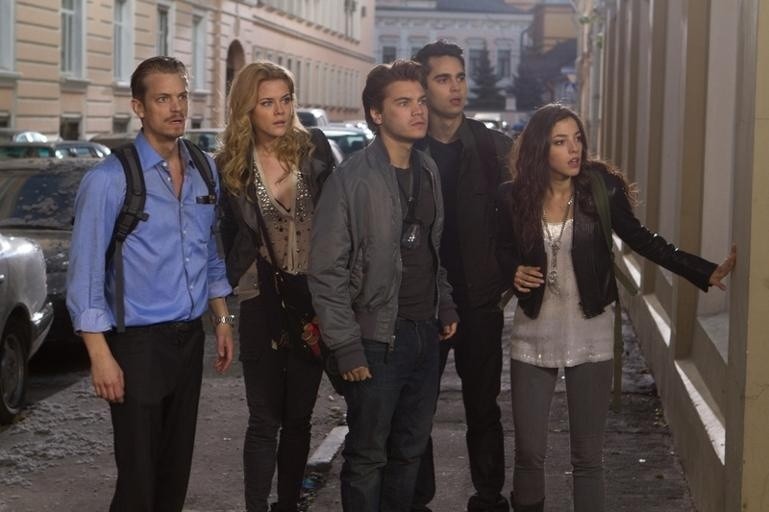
245 171 315 332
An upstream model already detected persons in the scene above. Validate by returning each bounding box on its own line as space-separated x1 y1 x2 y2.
308 59 458 512
215 61 335 512
412 41 515 511
66 56 233 512
494 103 736 512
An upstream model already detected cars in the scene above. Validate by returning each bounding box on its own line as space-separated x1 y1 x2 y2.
92 135 153 152
5 143 116 159
476 114 509 136
0 156 118 374
0 229 56 425
187 129 232 161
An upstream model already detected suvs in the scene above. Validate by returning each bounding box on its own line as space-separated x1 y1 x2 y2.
297 108 374 165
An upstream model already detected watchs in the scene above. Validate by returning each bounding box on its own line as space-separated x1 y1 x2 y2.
213 315 236 326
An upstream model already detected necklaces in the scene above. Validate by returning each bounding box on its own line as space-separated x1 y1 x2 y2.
537 192 572 297
384 150 424 254
